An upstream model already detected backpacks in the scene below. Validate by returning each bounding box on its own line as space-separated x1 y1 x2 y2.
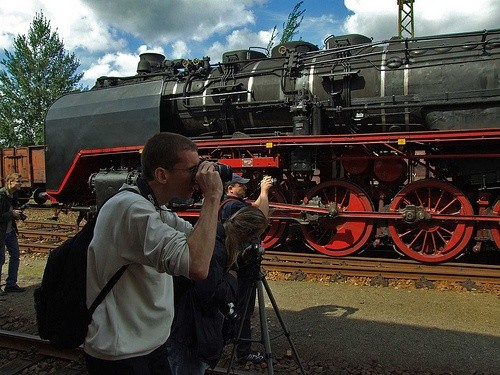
33 187 142 351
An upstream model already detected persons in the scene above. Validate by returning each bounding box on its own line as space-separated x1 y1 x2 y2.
221 174 272 362
167 206 267 375
83 132 222 375
0 173 25 296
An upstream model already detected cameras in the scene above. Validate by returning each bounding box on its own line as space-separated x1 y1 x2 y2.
199 158 233 182
263 176 277 187
18 212 27 221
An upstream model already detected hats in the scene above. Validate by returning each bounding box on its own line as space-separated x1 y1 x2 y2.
224 173 250 188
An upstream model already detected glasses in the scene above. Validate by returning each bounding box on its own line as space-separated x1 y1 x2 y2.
16 181 23 183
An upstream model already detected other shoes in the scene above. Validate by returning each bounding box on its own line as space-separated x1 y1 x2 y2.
4 284 24 292
236 350 265 365
0 289 5 296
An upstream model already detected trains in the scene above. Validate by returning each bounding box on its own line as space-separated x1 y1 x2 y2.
45 28 500 265
1 145 47 205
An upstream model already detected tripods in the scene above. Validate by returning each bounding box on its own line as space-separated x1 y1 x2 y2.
224 270 305 375
